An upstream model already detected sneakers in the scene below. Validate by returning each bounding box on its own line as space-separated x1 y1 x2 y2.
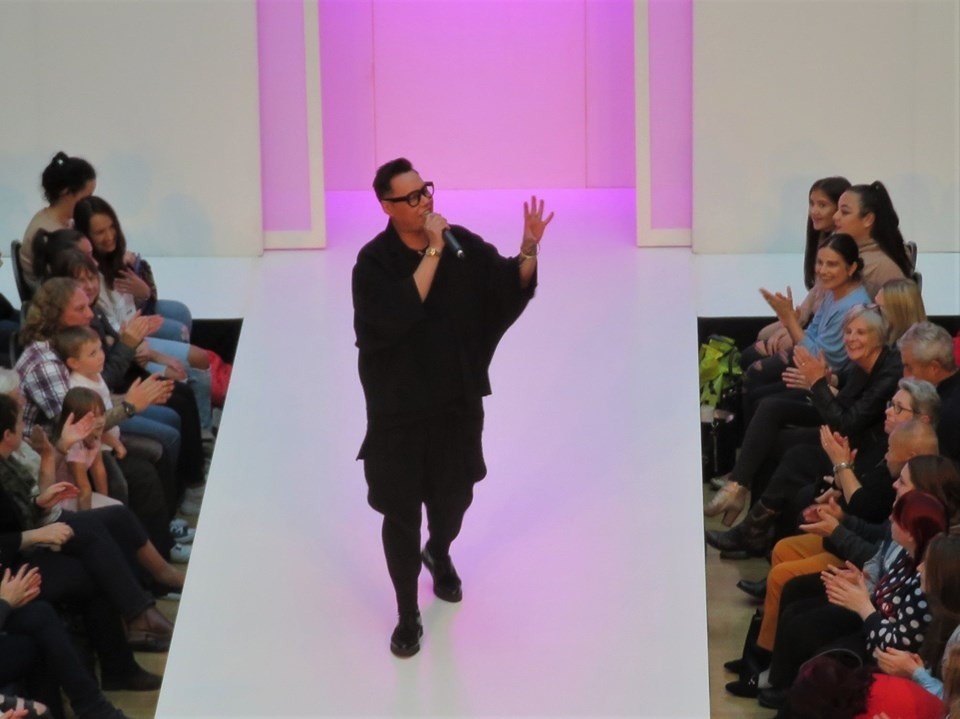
169 543 192 563
181 484 205 514
170 517 196 543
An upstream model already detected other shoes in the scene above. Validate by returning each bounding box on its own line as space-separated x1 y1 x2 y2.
150 577 183 595
126 618 175 644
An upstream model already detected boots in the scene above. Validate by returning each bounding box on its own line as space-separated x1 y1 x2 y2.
705 499 782 551
708 448 741 490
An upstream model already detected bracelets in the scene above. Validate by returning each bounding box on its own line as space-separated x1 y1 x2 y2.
520 243 541 259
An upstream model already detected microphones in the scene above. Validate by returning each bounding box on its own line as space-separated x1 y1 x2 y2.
424 213 466 261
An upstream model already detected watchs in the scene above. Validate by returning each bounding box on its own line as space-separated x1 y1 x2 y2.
426 247 441 258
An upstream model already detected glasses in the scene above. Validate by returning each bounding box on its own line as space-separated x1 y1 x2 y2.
382 181 435 208
886 400 921 415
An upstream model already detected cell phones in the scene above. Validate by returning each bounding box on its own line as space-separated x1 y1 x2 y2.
814 480 832 498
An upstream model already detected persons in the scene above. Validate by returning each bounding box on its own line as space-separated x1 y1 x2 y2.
0 151 220 719
351 157 555 659
704 176 960 719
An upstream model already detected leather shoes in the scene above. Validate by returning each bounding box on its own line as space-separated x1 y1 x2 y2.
735 577 767 600
422 541 463 603
390 612 423 658
758 687 789 708
101 668 164 690
724 659 742 674
725 679 762 698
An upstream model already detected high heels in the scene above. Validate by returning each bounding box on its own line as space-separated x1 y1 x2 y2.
704 480 750 527
